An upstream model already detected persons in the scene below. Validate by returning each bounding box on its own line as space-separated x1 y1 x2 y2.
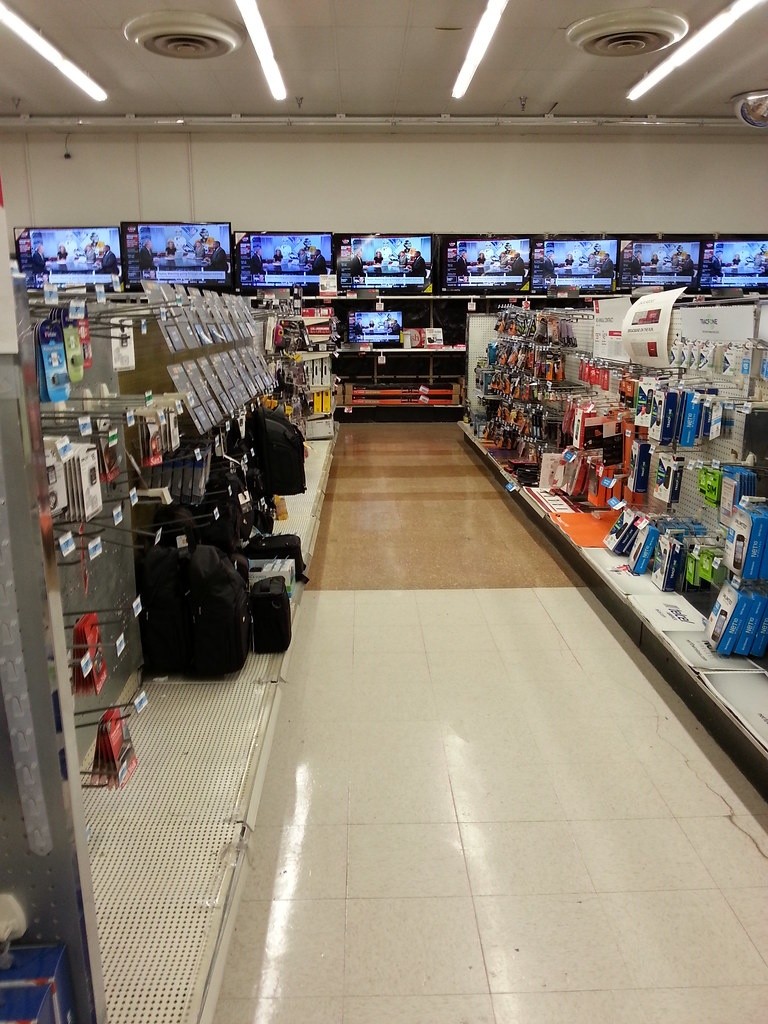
629 245 695 277
350 239 429 278
542 242 613 278
708 243 767 277
356 313 401 336
456 241 526 277
138 227 229 272
251 237 329 275
30 232 119 279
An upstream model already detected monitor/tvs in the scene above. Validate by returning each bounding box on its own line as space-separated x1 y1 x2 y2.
348 310 404 348
14 221 768 301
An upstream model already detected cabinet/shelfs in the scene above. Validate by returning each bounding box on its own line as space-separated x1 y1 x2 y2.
241 292 768 410
